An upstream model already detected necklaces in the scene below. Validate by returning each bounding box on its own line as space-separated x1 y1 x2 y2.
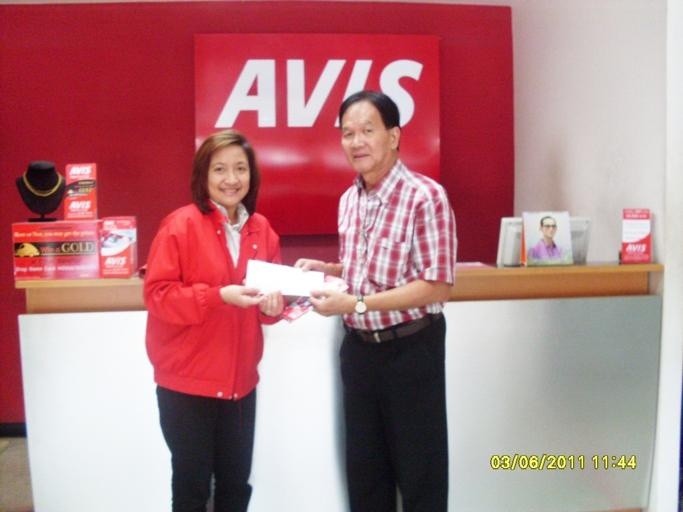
22 169 63 199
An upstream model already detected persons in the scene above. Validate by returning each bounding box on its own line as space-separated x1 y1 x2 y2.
292 90 457 512
526 215 563 261
141 129 283 511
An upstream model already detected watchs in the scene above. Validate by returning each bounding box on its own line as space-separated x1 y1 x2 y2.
355 296 366 317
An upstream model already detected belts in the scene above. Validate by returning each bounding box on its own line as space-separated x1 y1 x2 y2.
343 312 443 344
